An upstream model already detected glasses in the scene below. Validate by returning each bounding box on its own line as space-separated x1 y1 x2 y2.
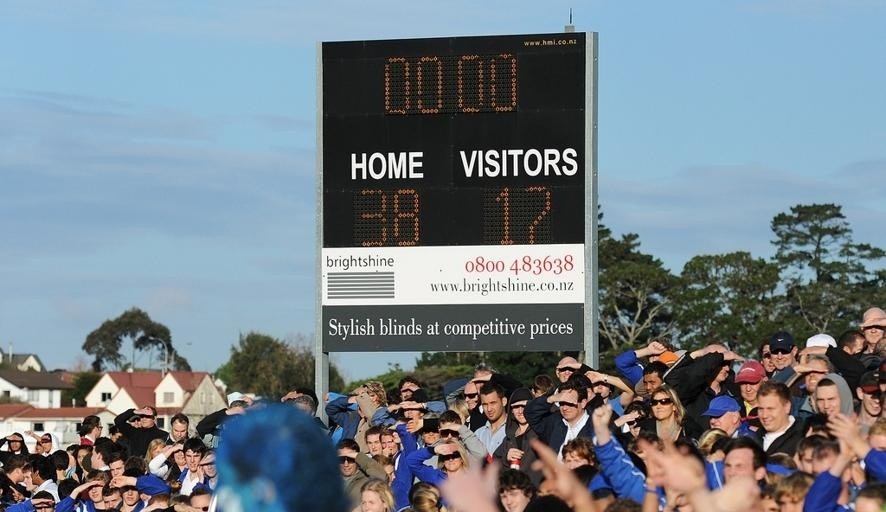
649 398 673 407
463 393 477 399
772 346 794 354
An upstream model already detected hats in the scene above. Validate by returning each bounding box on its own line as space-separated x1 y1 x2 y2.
733 361 766 384
40 434 50 443
701 396 739 417
510 389 531 407
769 331 793 353
859 371 880 393
